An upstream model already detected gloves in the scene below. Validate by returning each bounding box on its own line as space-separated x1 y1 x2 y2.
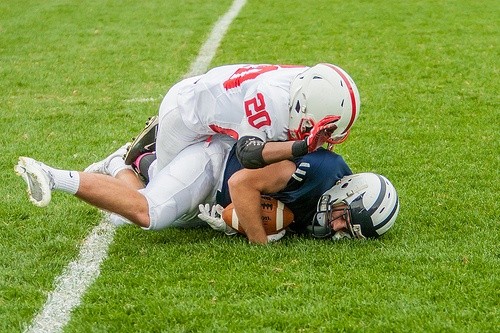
267 229 285 243
198 203 238 235
292 115 341 157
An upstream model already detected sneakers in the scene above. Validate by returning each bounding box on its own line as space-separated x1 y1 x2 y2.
14 156 56 208
83 142 131 174
122 115 159 180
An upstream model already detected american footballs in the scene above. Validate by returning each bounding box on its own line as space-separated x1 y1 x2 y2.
222 195 295 236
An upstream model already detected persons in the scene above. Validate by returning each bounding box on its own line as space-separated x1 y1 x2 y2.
122 62 360 184
13 135 401 244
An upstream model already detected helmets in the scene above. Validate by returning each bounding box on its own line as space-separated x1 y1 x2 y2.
311 173 400 240
289 63 360 151
